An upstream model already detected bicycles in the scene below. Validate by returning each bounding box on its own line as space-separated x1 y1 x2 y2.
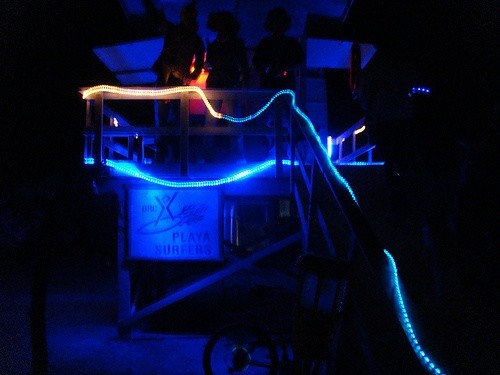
204 275 334 374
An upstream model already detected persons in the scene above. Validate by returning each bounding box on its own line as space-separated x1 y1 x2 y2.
252 7 304 160
206 12 247 162
143 0 205 163
